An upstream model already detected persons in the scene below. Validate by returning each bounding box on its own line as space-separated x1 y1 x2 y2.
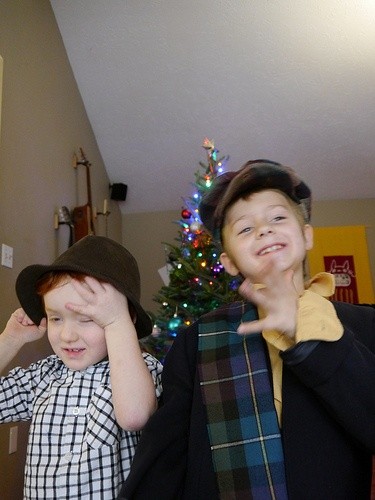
115 158 374 500
0 235 164 500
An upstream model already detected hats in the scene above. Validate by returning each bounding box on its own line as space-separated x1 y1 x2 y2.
199 159 311 252
15 235 153 341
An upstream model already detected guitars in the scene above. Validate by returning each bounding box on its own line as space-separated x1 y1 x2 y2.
68 147 96 249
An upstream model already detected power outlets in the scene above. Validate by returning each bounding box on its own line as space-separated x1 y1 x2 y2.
1 243 14 268
8 425 18 455
